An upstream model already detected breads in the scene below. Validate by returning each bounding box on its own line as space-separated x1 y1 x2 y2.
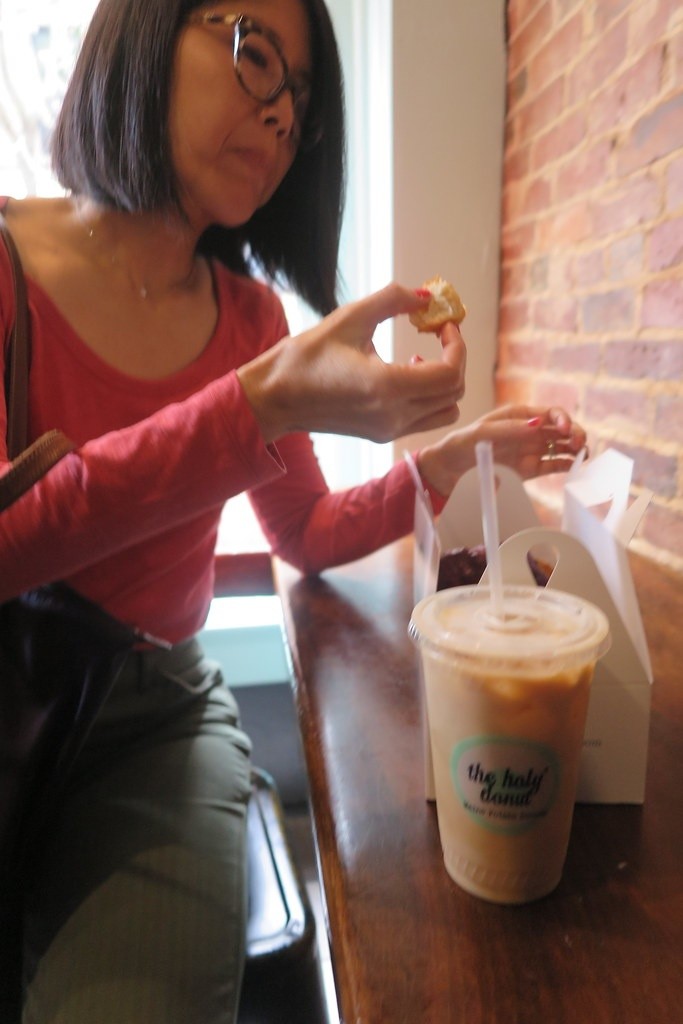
408 276 465 334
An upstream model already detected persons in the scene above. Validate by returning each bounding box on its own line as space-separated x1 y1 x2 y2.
0 1 590 1024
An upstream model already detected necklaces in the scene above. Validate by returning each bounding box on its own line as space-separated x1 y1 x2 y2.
75 203 197 300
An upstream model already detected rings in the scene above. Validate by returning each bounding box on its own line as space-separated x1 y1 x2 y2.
546 440 553 454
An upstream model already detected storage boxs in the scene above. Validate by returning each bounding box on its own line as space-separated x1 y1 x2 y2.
402 444 652 802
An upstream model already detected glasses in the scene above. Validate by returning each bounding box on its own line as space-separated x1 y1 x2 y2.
180 10 325 150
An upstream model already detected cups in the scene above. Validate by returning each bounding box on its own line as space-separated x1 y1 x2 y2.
407 583 611 905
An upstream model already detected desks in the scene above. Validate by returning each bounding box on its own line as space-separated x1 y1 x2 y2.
271 519 683 1024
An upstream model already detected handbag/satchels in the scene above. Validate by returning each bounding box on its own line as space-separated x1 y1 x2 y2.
0 215 140 865
